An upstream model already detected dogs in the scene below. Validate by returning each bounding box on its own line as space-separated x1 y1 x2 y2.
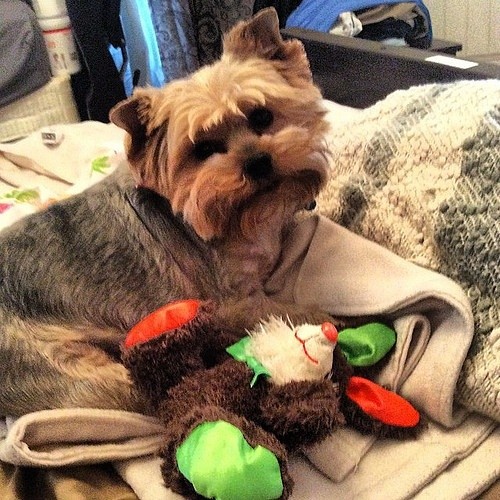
0 6 331 413
